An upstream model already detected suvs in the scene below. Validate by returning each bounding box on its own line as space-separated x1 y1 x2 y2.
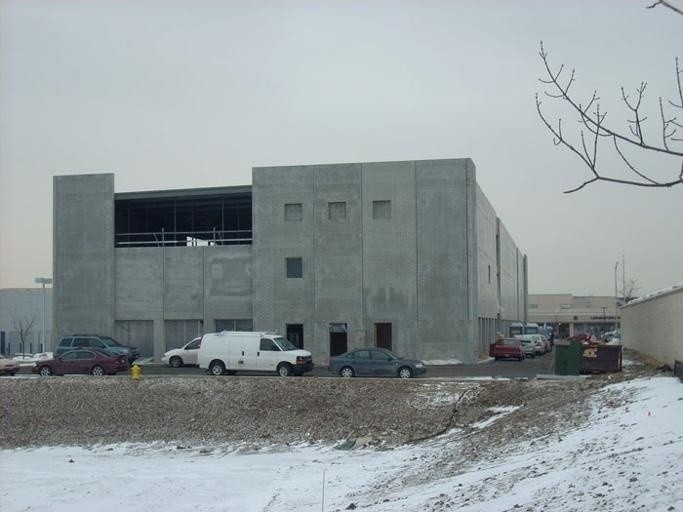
53 334 139 364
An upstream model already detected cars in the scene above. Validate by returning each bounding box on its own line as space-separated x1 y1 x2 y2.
489 334 552 362
161 336 202 368
567 331 620 342
0 351 52 376
327 346 427 379
32 349 129 377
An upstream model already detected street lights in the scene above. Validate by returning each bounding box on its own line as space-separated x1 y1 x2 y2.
602 307 608 333
34 277 52 352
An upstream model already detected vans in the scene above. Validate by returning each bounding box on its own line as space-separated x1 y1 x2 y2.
198 330 314 379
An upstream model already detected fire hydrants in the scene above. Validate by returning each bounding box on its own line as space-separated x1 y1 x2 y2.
130 364 142 380
